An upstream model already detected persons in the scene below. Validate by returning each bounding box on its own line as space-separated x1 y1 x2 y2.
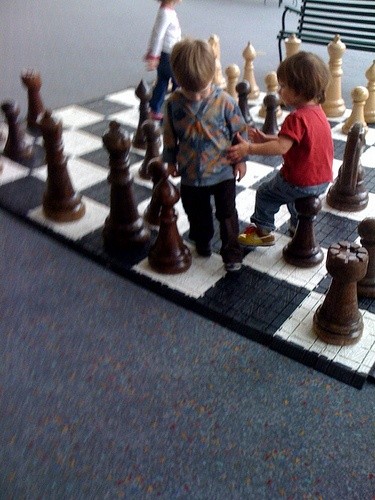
161 38 251 272
142 0 182 122
226 50 334 247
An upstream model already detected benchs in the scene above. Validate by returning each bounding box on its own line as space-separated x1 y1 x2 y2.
277 0 375 62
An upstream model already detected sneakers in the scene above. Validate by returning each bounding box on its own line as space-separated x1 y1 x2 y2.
238 226 275 246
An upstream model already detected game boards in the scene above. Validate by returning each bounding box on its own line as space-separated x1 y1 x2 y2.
1 34 375 392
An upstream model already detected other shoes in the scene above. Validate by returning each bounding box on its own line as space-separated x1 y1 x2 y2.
150 112 164 119
225 262 241 272
196 239 211 256
